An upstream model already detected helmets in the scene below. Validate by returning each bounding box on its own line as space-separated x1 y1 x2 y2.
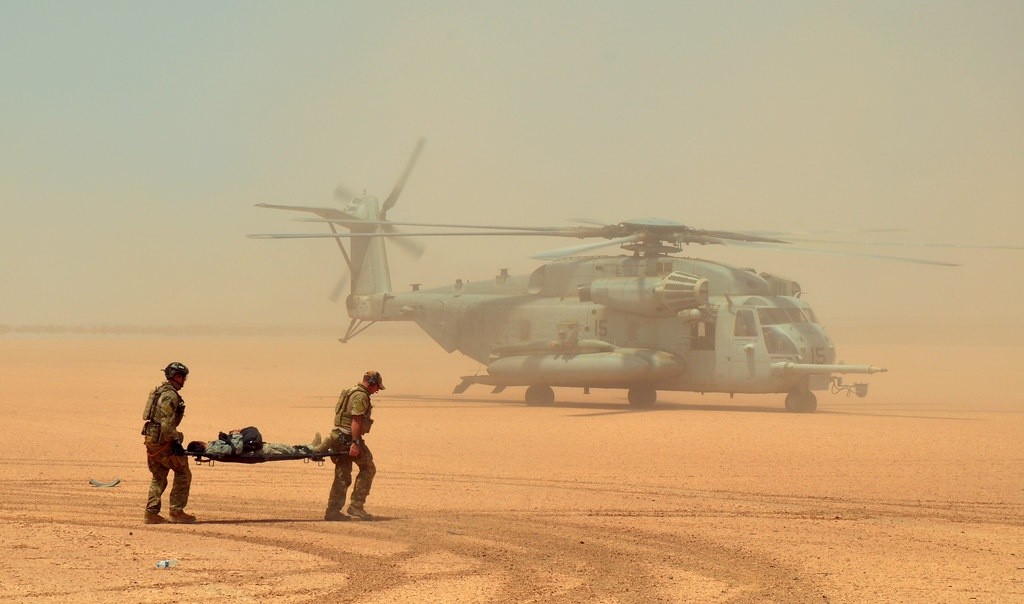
164 362 189 379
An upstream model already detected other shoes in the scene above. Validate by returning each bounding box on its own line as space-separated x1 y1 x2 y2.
325 508 351 521
347 504 373 519
144 512 171 525
168 510 196 522
311 432 332 453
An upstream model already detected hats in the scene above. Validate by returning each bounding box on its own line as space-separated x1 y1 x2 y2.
364 371 385 390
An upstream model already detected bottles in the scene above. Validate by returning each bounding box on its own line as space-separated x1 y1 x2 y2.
156 560 178 568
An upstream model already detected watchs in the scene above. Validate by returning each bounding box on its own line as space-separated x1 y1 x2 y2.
352 438 363 445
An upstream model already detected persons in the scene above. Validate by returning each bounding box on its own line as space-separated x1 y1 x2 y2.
324 370 386 521
188 428 332 462
141 361 196 524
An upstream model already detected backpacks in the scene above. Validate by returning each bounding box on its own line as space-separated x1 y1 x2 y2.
218 426 263 454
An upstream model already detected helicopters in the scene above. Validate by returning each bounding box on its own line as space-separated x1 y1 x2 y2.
243 138 992 415
